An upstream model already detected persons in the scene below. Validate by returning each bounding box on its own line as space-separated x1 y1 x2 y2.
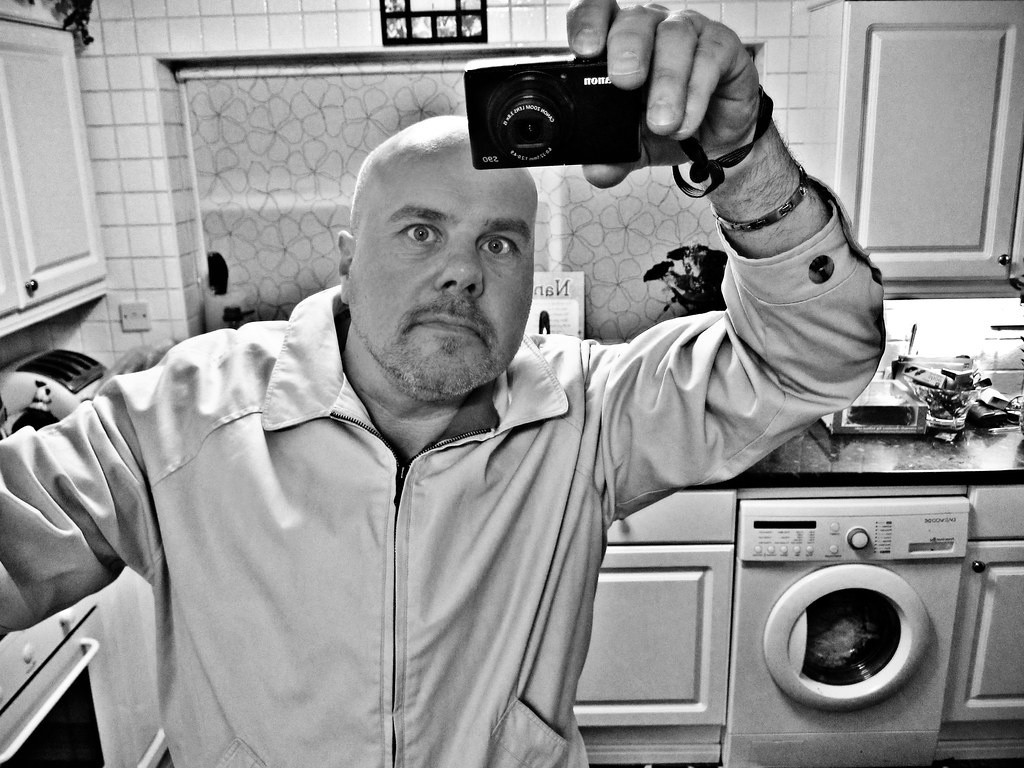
0 0 886 768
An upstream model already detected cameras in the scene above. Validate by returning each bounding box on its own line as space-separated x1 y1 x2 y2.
464 54 643 171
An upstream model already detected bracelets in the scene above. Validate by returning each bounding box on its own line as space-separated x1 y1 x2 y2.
710 164 808 233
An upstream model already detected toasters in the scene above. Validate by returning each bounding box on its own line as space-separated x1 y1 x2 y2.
0 346 109 419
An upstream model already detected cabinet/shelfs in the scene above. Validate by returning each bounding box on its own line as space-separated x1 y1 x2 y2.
803 0 1024 298
1 15 105 340
939 484 1024 723
571 491 738 729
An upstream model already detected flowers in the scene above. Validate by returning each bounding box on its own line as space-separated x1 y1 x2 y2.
644 246 729 316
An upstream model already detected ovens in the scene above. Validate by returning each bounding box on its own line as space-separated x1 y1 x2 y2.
0 596 136 768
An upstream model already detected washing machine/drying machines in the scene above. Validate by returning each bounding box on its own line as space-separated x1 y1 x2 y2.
721 497 971 767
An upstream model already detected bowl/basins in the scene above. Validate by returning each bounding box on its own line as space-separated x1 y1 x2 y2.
904 373 988 430
